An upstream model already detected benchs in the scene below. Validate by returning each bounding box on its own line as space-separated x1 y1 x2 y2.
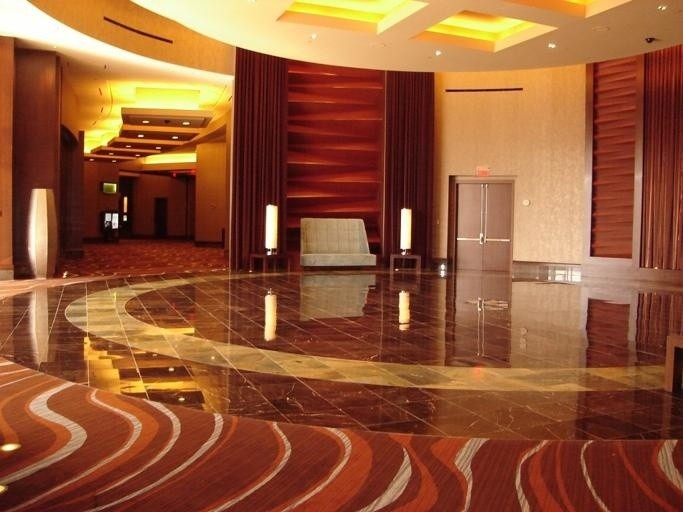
299 217 377 272
299 273 377 322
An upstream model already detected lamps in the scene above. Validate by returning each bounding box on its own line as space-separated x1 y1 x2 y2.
400 207 413 256
265 204 278 255
84 107 214 164
398 290 411 332
264 287 278 342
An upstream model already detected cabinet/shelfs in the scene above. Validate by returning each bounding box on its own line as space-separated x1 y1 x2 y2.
390 253 421 272
249 253 293 274
